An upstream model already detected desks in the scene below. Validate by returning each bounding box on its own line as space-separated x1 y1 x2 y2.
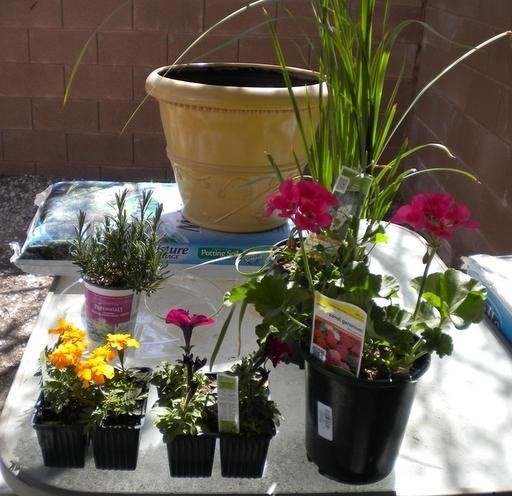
0 219 512 496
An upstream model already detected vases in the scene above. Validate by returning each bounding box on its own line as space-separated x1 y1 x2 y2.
218 432 273 479
90 412 144 471
299 323 432 484
29 405 94 470
145 61 330 234
166 432 218 478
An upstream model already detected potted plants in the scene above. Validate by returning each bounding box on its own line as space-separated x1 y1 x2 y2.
65 187 170 344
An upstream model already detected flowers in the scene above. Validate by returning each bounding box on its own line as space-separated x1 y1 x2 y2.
89 333 153 415
147 309 216 442
210 331 293 431
32 317 113 420
220 177 487 375
